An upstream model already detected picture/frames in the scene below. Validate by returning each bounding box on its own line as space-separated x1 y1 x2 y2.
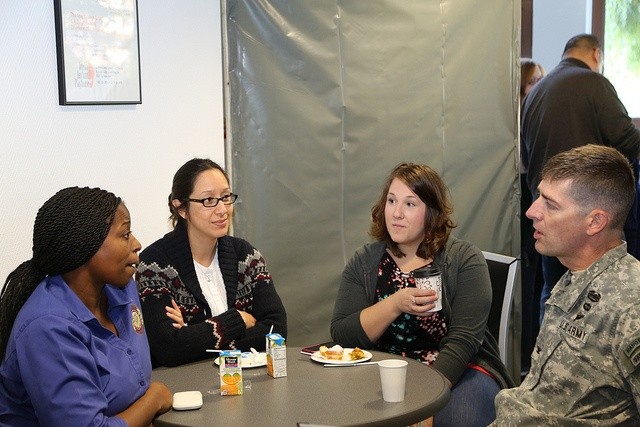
54 0 142 105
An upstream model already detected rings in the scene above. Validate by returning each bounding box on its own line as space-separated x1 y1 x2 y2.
412 296 415 303
412 296 416 303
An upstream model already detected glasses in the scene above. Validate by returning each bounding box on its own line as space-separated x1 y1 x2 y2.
189 192 238 208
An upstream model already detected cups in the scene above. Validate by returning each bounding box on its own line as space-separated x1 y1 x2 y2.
377 358 409 402
413 267 444 312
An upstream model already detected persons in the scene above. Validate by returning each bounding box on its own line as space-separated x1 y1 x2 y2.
331 162 514 426
135 159 288 366
489 144 640 425
521 33 640 323
521 62 542 375
1 186 173 425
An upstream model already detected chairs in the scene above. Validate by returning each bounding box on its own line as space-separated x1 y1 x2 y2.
480 249 520 367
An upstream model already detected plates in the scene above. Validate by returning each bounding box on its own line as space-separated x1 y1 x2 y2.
212 350 268 370
310 348 372 364
